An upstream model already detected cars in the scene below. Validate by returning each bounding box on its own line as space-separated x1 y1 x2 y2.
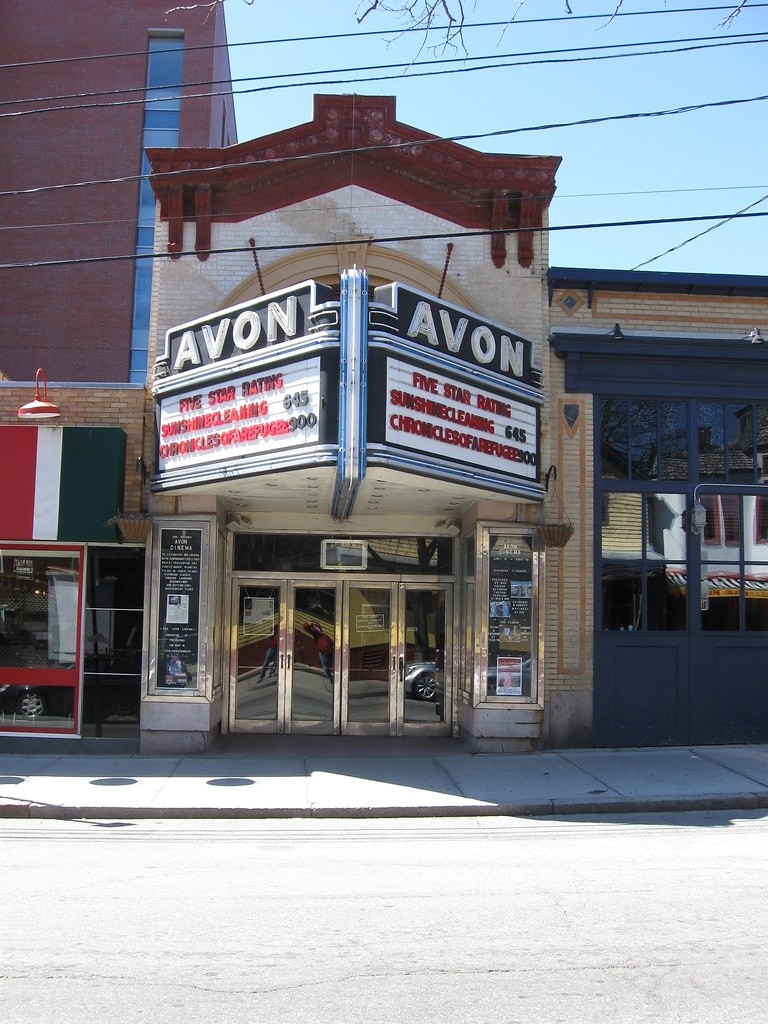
488 652 531 696
0 654 142 724
404 662 436 702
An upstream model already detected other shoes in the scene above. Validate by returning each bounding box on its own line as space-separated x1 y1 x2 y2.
256 678 262 684
268 672 271 678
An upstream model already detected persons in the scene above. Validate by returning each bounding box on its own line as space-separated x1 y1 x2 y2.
264 601 273 621
256 621 278 684
301 618 333 689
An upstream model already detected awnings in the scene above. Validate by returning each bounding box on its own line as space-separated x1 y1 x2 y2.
665 567 768 600
